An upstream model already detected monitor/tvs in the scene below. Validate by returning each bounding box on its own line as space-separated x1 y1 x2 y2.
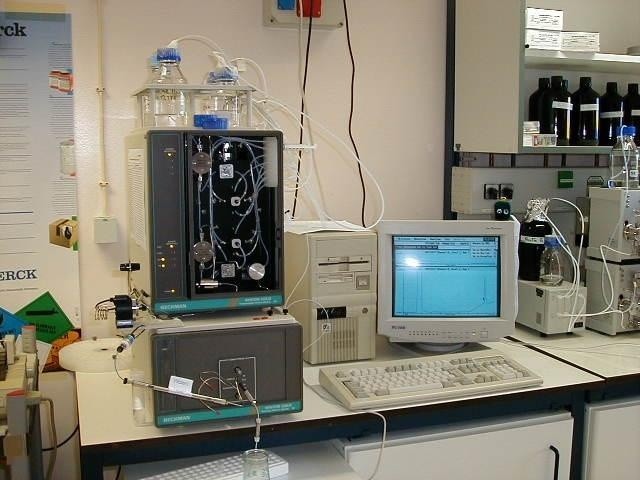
377 219 520 357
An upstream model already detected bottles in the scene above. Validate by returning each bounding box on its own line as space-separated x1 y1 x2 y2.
610 125 639 191
200 67 245 129
528 74 640 146
130 372 155 427
538 234 565 288
141 47 194 128
518 198 554 282
242 447 270 480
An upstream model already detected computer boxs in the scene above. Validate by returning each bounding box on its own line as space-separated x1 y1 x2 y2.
285 221 378 366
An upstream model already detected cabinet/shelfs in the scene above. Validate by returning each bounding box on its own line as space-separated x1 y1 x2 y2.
454 0 639 154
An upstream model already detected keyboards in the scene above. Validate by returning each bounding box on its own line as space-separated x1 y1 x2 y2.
319 349 545 411
139 449 288 480
